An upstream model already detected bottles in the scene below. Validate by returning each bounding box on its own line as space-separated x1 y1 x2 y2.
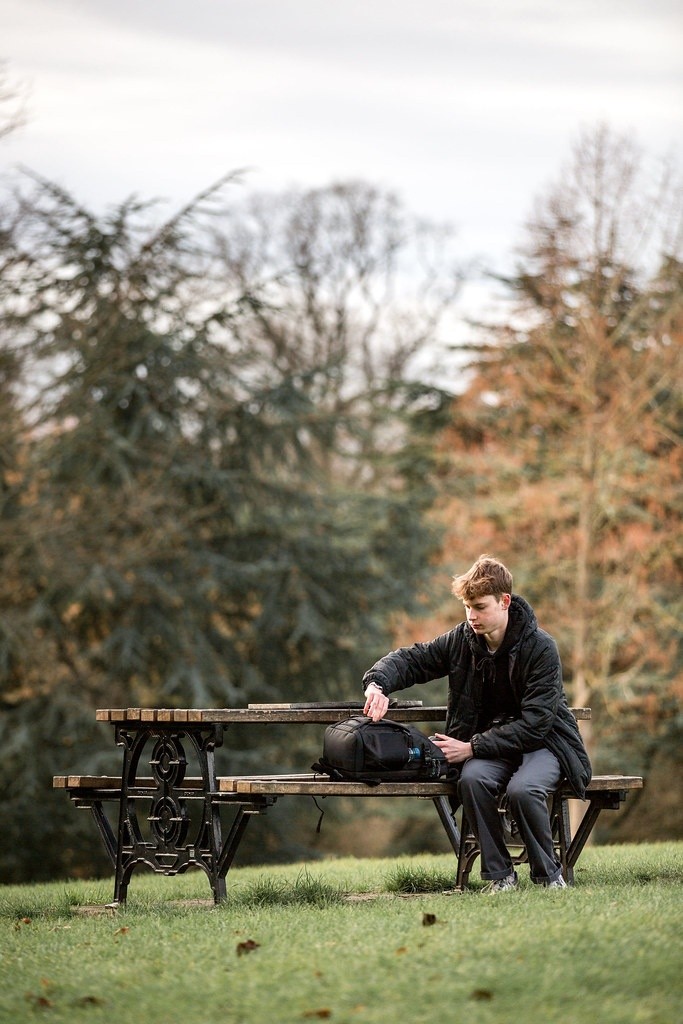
407 748 420 761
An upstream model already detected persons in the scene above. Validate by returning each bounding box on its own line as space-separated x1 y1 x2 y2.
360 554 593 896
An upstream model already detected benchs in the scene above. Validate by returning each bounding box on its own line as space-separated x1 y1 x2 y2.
54 772 325 812
218 775 644 811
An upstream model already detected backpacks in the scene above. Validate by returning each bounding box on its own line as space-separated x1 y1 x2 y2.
311 714 459 786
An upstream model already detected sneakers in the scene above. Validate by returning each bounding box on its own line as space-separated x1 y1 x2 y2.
481 871 518 896
542 875 567 889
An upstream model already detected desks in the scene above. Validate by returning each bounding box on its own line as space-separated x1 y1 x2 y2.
95 707 592 911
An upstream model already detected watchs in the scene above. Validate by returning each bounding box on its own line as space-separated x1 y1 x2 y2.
370 682 383 691
